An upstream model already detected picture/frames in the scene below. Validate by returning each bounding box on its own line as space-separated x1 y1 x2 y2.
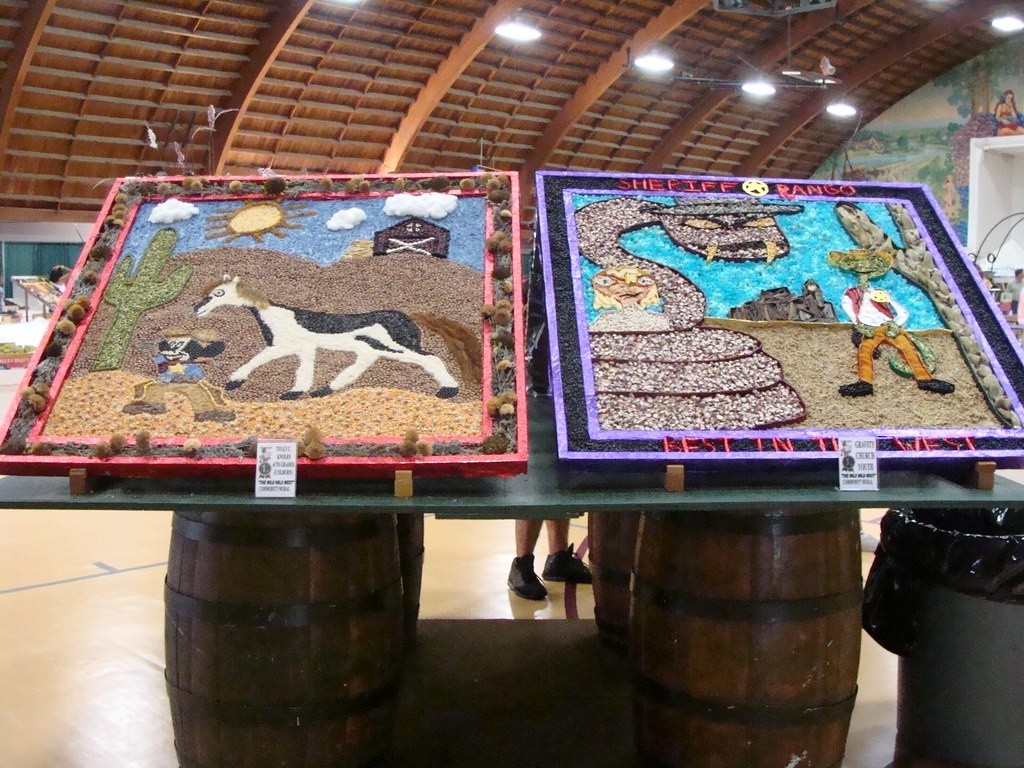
0 171 530 478
535 170 1024 470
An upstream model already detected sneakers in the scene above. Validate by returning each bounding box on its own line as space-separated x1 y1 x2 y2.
507 554 548 600
542 542 593 583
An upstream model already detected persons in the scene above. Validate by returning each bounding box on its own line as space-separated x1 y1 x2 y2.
49 265 63 282
1000 269 1024 313
507 519 593 600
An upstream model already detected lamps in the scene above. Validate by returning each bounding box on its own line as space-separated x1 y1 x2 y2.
634 45 674 71
315 0 366 9
495 15 542 43
826 98 857 118
741 74 776 95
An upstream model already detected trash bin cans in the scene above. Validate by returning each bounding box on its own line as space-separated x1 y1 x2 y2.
880 505 1024 768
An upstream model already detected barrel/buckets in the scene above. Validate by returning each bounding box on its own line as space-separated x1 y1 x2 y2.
589 512 862 768
165 513 424 768
